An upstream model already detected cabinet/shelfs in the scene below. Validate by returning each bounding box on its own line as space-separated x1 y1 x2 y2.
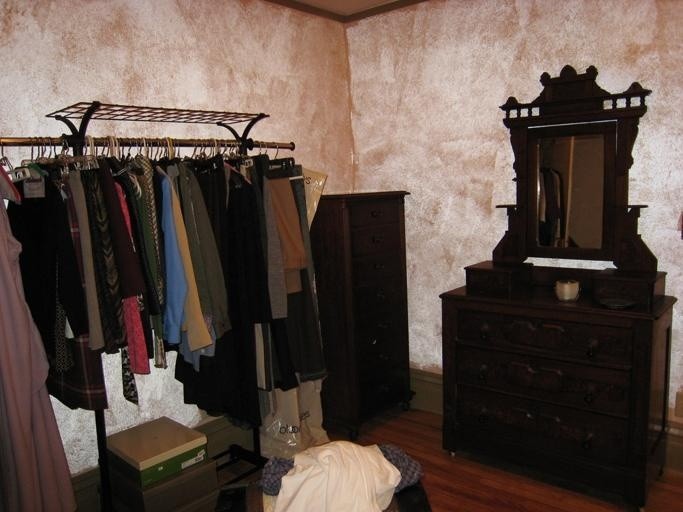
438 260 677 510
310 190 417 442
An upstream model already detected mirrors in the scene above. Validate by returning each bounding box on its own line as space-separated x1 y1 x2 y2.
492 63 658 273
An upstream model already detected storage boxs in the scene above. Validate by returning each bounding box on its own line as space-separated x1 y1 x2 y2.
111 461 218 511
107 416 208 486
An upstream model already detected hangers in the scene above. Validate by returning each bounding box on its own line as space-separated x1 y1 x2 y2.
1 136 282 169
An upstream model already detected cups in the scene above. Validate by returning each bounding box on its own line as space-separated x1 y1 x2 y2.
556 279 579 301
